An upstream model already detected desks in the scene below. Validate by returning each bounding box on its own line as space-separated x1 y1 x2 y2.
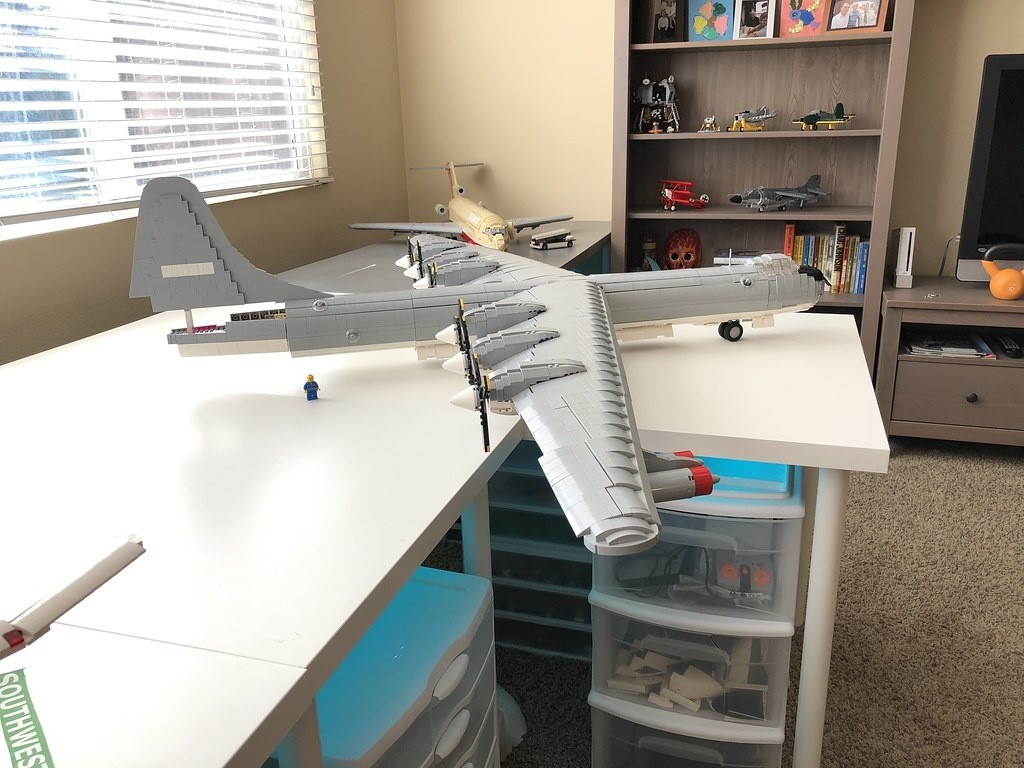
0 301 529 768
271 220 613 307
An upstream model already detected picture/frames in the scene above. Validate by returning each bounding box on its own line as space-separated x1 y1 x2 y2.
820 0 889 36
732 0 776 40
643 0 688 43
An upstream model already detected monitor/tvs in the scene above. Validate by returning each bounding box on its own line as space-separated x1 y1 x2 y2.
955 53 1024 281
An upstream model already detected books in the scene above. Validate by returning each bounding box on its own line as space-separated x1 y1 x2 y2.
784 222 869 295
900 328 996 360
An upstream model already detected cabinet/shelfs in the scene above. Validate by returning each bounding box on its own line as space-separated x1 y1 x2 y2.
875 276 1024 453
588 314 893 768
611 0 917 384
270 565 501 768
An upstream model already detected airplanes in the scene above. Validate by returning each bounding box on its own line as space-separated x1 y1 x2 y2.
347 160 575 252
655 178 710 212
127 176 830 557
726 175 833 215
791 102 858 131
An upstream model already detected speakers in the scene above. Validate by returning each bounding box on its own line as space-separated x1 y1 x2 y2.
887 227 916 289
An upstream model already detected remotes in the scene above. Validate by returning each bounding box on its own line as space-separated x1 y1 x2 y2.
994 334 1022 358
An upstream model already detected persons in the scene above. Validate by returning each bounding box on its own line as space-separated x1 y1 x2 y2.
742 9 768 38
831 0 876 29
658 1 676 38
